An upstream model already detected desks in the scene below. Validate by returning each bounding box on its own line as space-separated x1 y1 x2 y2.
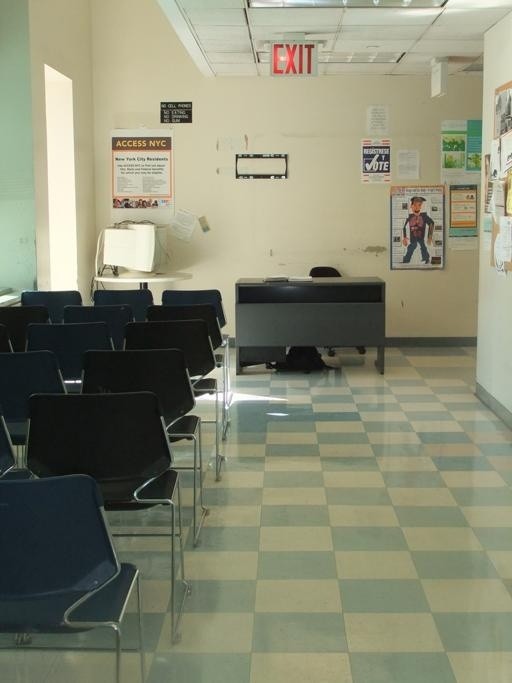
93 274 194 291
235 276 386 375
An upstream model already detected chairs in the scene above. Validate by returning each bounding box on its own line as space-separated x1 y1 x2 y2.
1 418 36 480
81 350 211 545
1 327 19 470
309 266 366 356
161 289 231 410
61 305 136 349
94 289 154 323
2 465 144 683
29 392 195 645
153 307 231 444
26 322 111 462
3 308 50 351
122 320 228 482
21 291 77 324
0 353 68 468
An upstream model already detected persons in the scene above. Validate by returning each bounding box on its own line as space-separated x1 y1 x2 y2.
403 197 434 263
113 198 158 208
